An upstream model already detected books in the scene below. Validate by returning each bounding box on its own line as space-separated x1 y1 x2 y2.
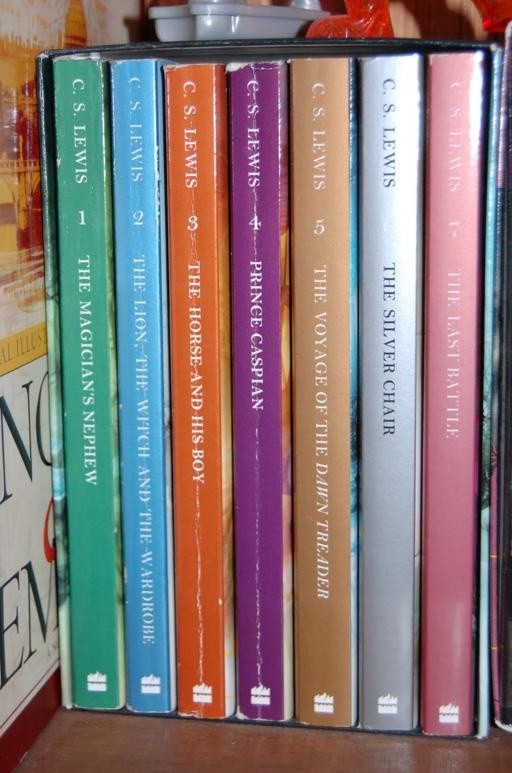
48 50 483 740
